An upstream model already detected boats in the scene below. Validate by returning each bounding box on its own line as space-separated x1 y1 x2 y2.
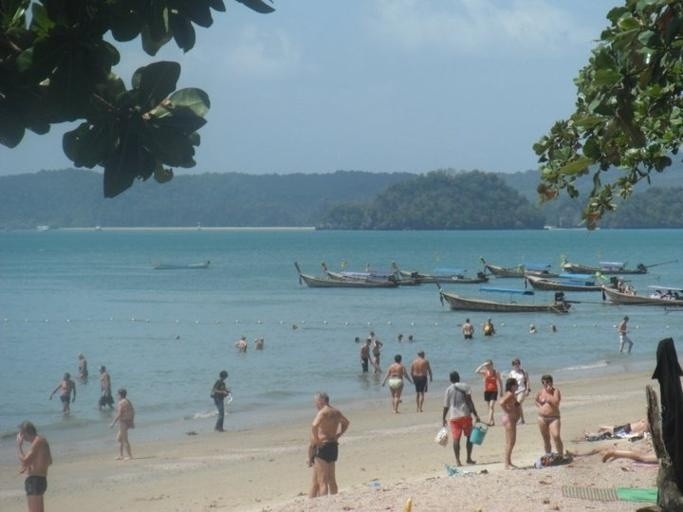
433 278 571 314
151 257 211 269
599 283 683 306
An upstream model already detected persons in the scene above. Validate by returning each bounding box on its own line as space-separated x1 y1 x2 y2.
529 324 536 333
98 365 115 410
382 354 411 413
598 421 657 463
14 420 52 512
234 335 264 352
307 392 349 497
50 372 76 413
397 334 413 343
483 318 495 336
463 318 474 339
619 317 633 352
212 371 229 432
77 353 88 377
442 359 562 469
360 331 383 373
111 388 134 460
410 351 432 413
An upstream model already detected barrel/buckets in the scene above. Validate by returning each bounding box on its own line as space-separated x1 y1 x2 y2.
468 418 488 446
